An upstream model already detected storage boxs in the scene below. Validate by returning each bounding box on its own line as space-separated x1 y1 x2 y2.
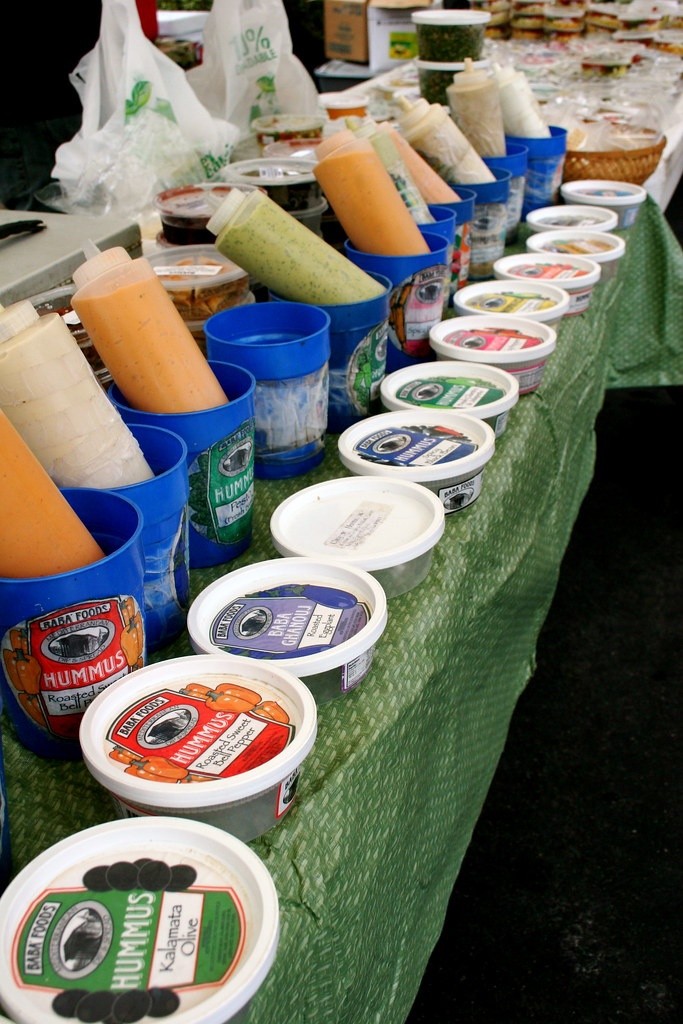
323 0 444 70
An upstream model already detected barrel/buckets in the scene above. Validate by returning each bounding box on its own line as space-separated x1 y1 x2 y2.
0 123 566 763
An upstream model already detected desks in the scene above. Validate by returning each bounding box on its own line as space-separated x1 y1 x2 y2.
1 114 682 1024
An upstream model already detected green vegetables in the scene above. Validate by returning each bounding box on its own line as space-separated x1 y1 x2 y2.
413 24 484 106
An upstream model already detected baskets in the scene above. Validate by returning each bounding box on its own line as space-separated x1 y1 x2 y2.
562 120 667 187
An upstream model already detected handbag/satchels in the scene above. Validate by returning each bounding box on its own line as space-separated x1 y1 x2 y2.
185 0 329 163
49 0 241 221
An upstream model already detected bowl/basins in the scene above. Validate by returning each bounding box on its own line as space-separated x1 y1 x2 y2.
59 0 682 391
1 179 648 1023
